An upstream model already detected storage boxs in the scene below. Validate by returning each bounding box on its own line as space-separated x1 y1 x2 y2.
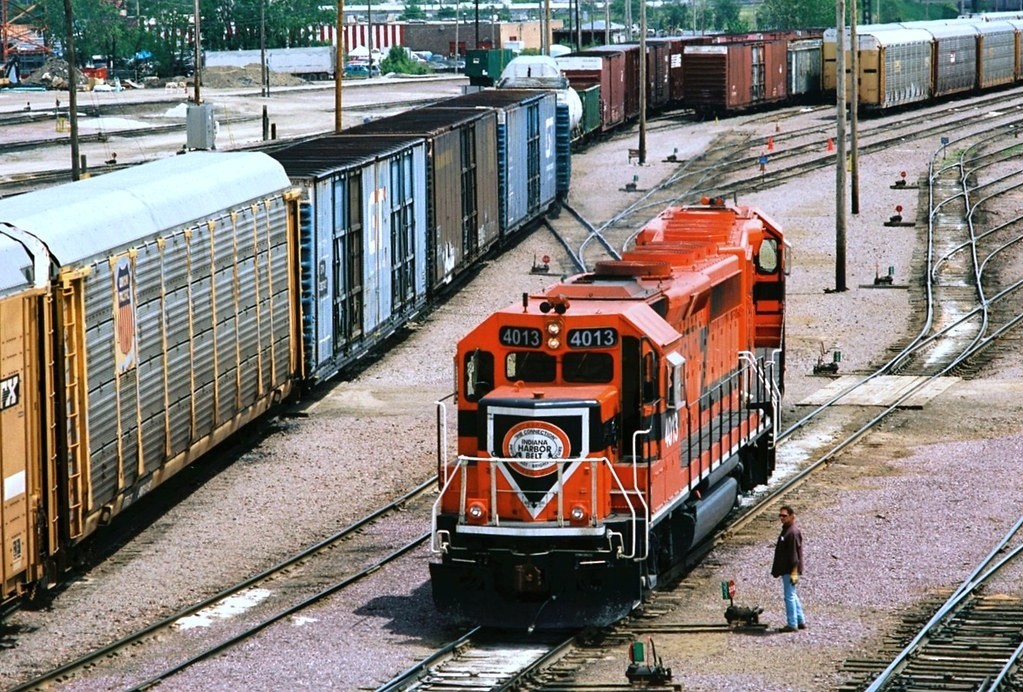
269 28 821 386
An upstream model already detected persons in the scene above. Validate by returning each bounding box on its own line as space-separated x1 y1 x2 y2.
2 47 22 92
772 505 808 633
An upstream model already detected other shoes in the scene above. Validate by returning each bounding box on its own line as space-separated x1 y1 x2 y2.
779 626 798 632
784 624 805 629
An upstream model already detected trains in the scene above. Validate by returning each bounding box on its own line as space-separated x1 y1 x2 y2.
0 30 821 609
676 40 816 117
426 197 796 610
820 15 1022 113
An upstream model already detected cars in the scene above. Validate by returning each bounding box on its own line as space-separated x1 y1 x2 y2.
340 46 465 77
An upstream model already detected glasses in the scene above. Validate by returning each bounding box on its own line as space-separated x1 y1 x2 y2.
779 514 790 518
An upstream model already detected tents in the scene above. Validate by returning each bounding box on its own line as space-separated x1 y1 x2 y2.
347 43 376 63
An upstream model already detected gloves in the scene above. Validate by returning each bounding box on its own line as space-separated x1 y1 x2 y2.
790 565 798 585
